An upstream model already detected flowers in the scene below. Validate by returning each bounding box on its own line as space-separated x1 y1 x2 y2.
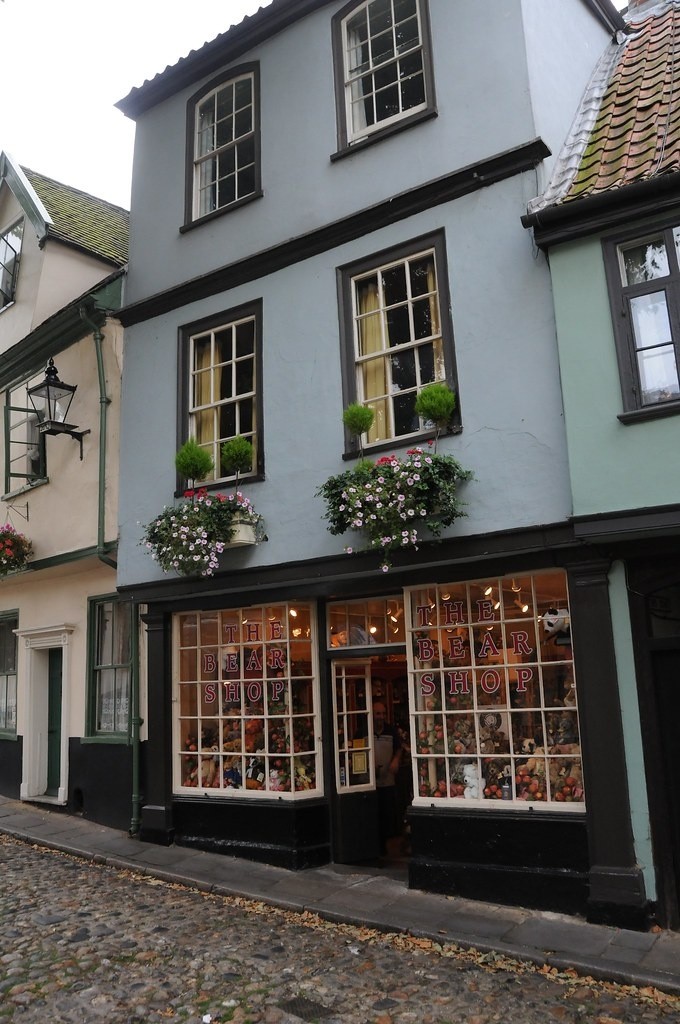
0 523 32 577
317 383 470 579
136 434 261 582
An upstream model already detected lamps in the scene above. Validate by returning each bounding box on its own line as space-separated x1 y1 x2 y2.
289 608 300 617
238 614 247 624
513 593 529 612
388 624 399 633
267 607 276 620
490 599 500 609
427 589 435 609
428 614 437 626
480 585 492 596
511 577 521 592
390 608 403 622
440 588 450 600
369 625 377 633
26 356 90 461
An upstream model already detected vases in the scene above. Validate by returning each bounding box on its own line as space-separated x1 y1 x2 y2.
214 512 258 548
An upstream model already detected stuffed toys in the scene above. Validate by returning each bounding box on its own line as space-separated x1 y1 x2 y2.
396 726 407 739
455 712 582 799
543 607 571 638
458 628 499 656
331 632 347 647
357 689 363 697
190 703 314 790
221 642 292 671
373 681 382 697
393 687 401 703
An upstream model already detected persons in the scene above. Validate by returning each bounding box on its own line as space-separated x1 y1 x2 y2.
401 744 414 825
355 701 402 856
554 646 574 705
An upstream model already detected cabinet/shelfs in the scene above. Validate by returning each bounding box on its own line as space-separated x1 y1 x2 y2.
294 659 407 741
